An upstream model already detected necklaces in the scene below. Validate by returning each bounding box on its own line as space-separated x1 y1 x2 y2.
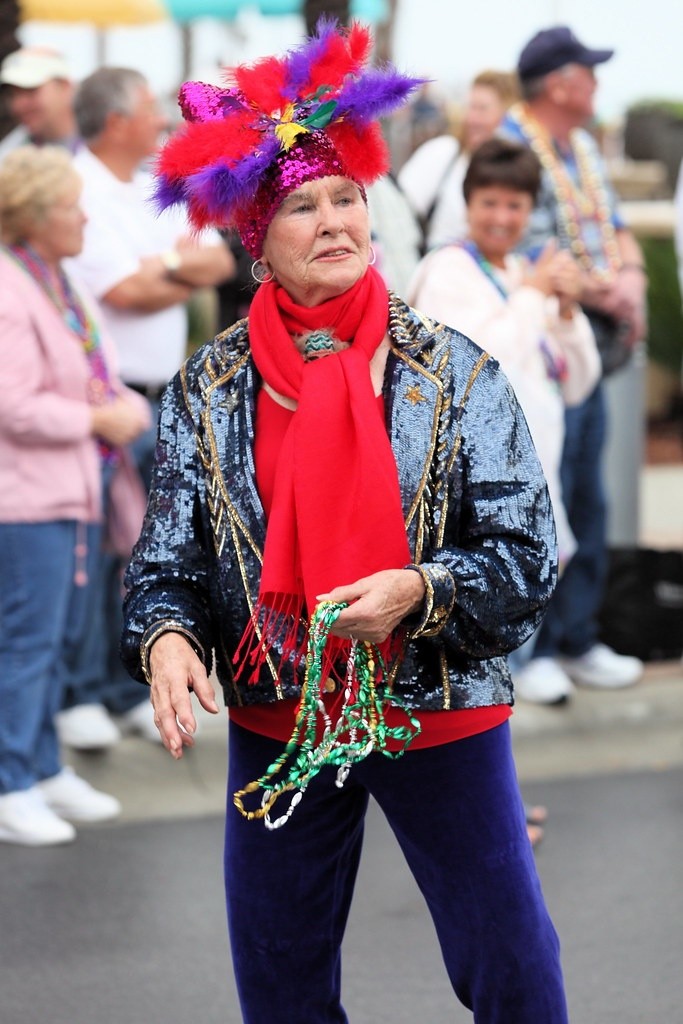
234 603 421 828
508 103 621 281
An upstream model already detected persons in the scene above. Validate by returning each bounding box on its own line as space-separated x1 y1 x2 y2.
122 19 569 1024
0 26 648 846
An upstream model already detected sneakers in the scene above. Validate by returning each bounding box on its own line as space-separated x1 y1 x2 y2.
59 701 120 756
0 787 78 852
510 654 576 704
129 699 207 749
42 766 125 825
553 643 643 691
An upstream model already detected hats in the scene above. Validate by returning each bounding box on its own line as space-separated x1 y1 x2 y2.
155 16 434 257
0 44 75 87
515 22 617 80
